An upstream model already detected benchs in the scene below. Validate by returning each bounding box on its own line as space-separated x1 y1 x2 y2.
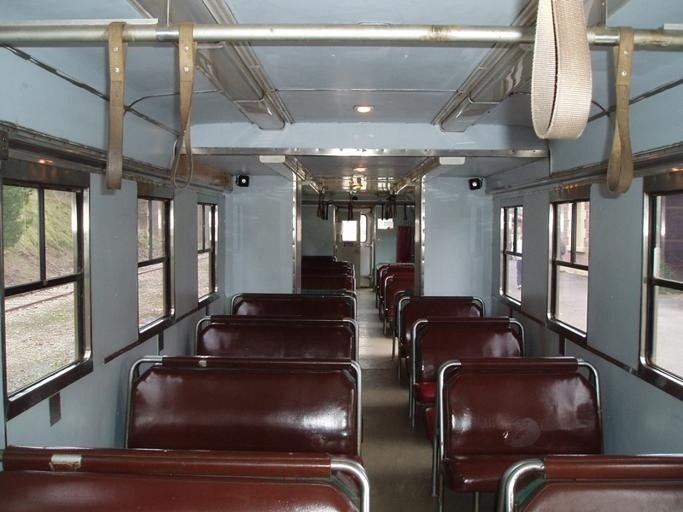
1 253 369 512
375 263 682 512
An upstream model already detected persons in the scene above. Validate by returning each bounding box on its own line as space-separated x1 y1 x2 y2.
510 237 522 289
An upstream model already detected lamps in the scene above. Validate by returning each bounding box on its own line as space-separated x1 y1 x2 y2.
470 178 484 190
354 104 375 114
235 174 250 188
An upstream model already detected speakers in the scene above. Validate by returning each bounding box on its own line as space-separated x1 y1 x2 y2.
236 175 249 187
469 178 481 190
